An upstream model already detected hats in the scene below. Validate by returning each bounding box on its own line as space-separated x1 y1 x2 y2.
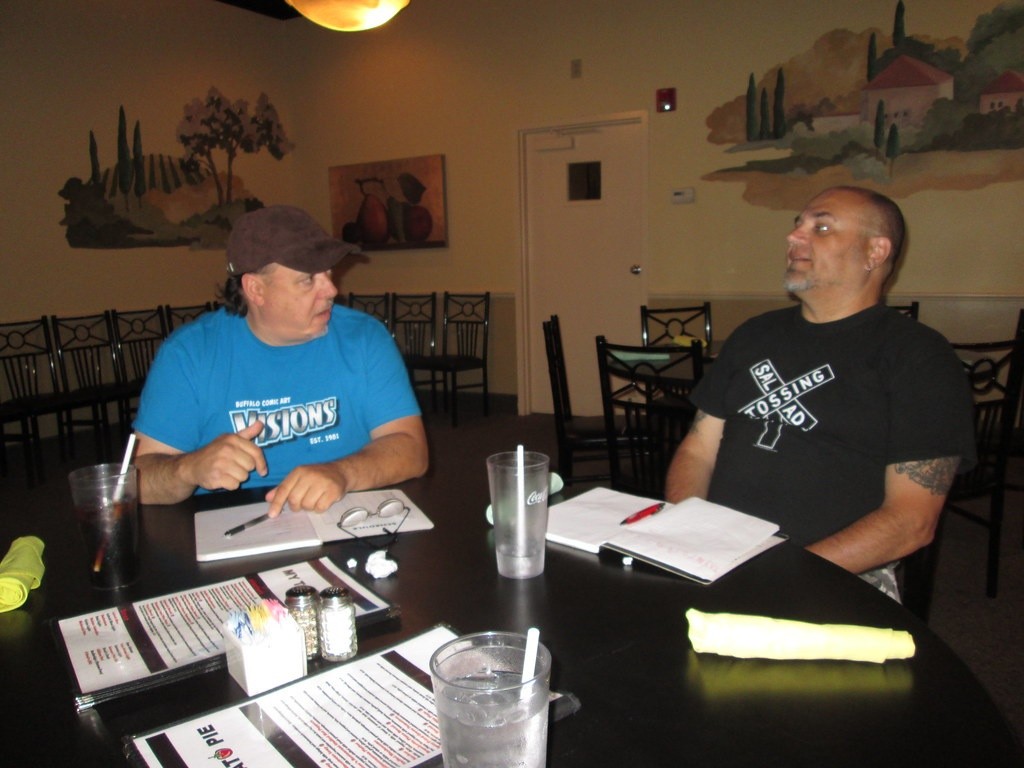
225 205 353 276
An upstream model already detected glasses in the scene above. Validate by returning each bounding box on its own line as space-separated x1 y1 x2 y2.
338 498 411 550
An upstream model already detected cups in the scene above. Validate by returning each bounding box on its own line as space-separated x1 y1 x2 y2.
487 452 549 579
69 463 137 591
430 631 552 768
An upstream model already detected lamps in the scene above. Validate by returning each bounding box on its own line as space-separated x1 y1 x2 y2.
282 0 410 32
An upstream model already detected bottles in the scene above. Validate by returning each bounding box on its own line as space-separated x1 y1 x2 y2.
285 586 320 660
318 587 358 662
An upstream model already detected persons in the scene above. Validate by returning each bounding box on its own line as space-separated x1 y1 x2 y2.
665 184 976 602
132 208 430 516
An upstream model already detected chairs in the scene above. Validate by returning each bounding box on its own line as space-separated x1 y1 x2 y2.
333 291 489 428
542 302 1022 596
0 301 226 487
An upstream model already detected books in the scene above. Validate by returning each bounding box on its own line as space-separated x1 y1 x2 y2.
510 486 790 585
194 489 434 561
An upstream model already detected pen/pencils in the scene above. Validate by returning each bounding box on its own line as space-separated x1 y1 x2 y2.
619 502 666 524
225 514 267 540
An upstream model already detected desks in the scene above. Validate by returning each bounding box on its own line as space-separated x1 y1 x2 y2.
0 475 1023 767
607 340 725 502
